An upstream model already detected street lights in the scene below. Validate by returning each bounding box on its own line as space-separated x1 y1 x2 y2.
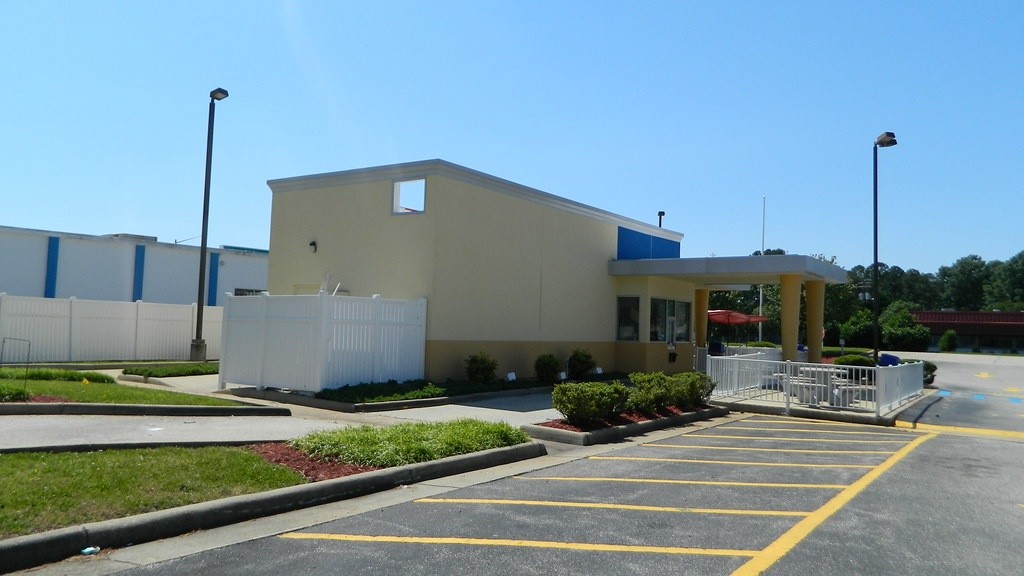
872 131 898 365
657 211 665 228
189 88 229 362
758 195 766 342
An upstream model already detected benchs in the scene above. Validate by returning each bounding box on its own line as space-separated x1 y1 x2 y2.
774 373 876 407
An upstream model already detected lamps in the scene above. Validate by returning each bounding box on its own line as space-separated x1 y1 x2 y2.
309 241 317 254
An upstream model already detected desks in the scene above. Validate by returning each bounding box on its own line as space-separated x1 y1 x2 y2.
801 367 849 401
